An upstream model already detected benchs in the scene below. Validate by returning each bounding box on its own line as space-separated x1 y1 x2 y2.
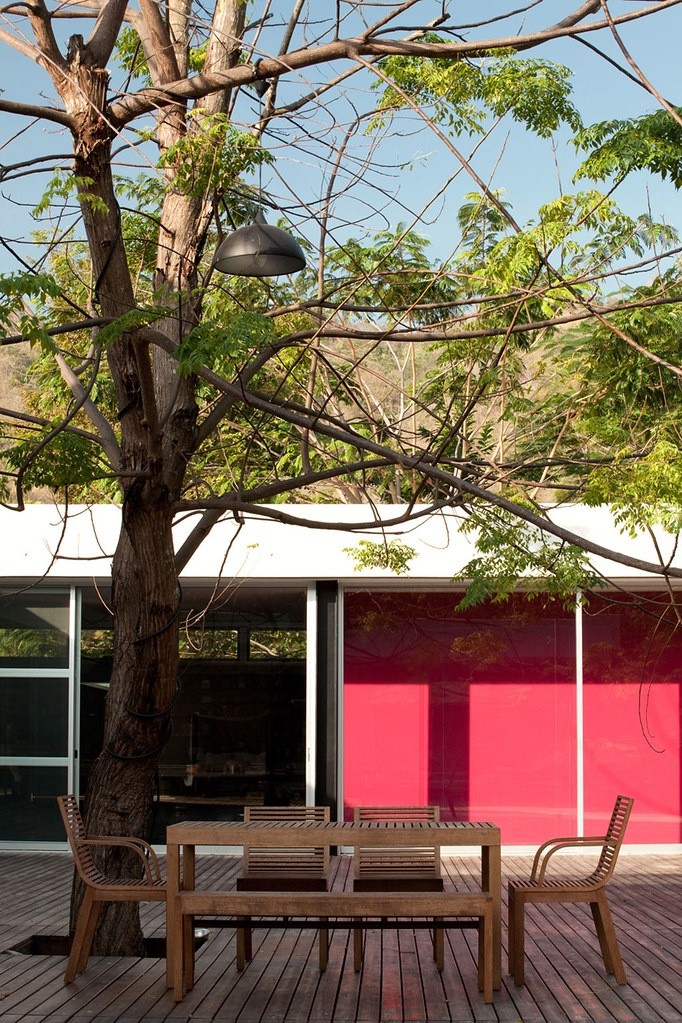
173 893 493 1002
152 794 265 806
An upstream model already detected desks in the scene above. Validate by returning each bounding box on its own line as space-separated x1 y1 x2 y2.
157 771 268 778
166 821 501 990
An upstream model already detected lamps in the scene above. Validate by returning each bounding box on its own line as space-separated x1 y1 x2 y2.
213 58 306 277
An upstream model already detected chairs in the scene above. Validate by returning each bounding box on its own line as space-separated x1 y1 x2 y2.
352 806 443 973
508 795 634 986
58 794 186 988
236 807 330 973
